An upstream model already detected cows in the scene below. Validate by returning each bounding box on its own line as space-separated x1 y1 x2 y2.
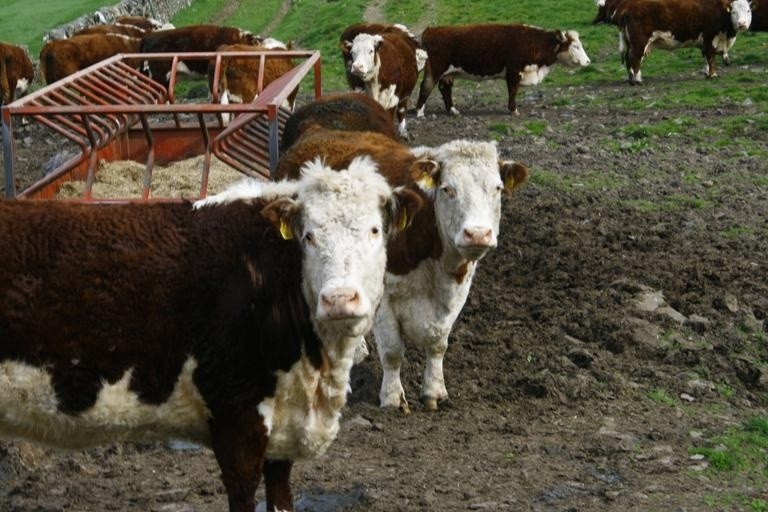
0 43 34 126
279 92 403 158
593 0 768 84
38 16 299 129
415 22 591 122
0 156 423 512
274 123 529 414
339 22 428 141
